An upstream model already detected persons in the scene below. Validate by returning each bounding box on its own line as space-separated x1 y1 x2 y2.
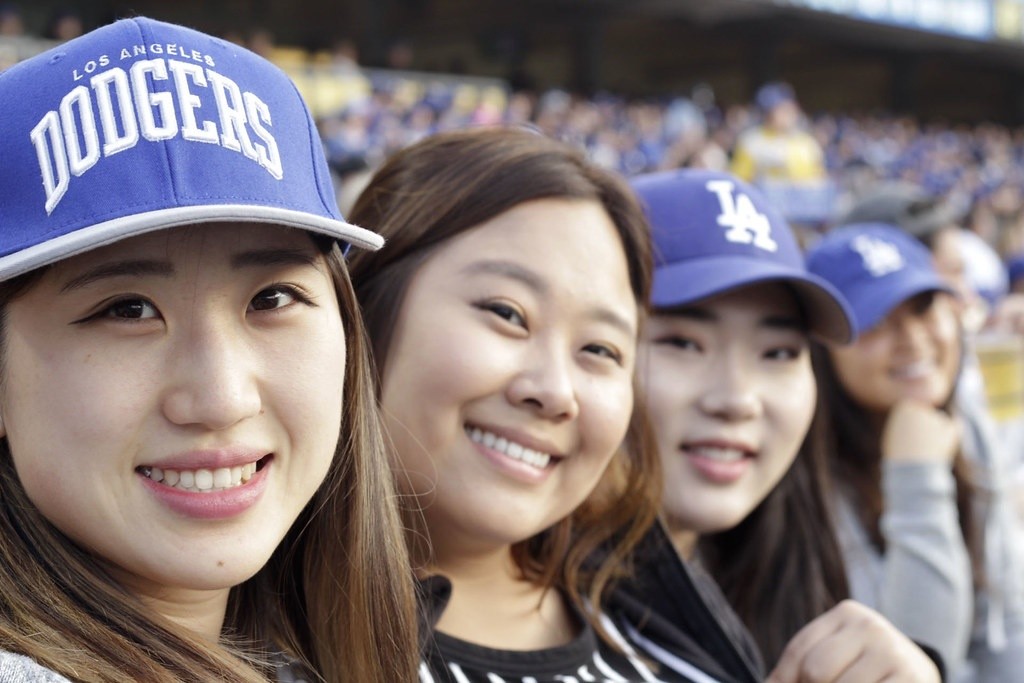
626 171 954 683
0 10 1021 337
803 219 1024 683
345 123 740 682
0 16 423 683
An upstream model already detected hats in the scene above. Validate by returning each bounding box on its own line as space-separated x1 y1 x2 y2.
2 16 384 291
809 220 966 341
624 168 856 348
